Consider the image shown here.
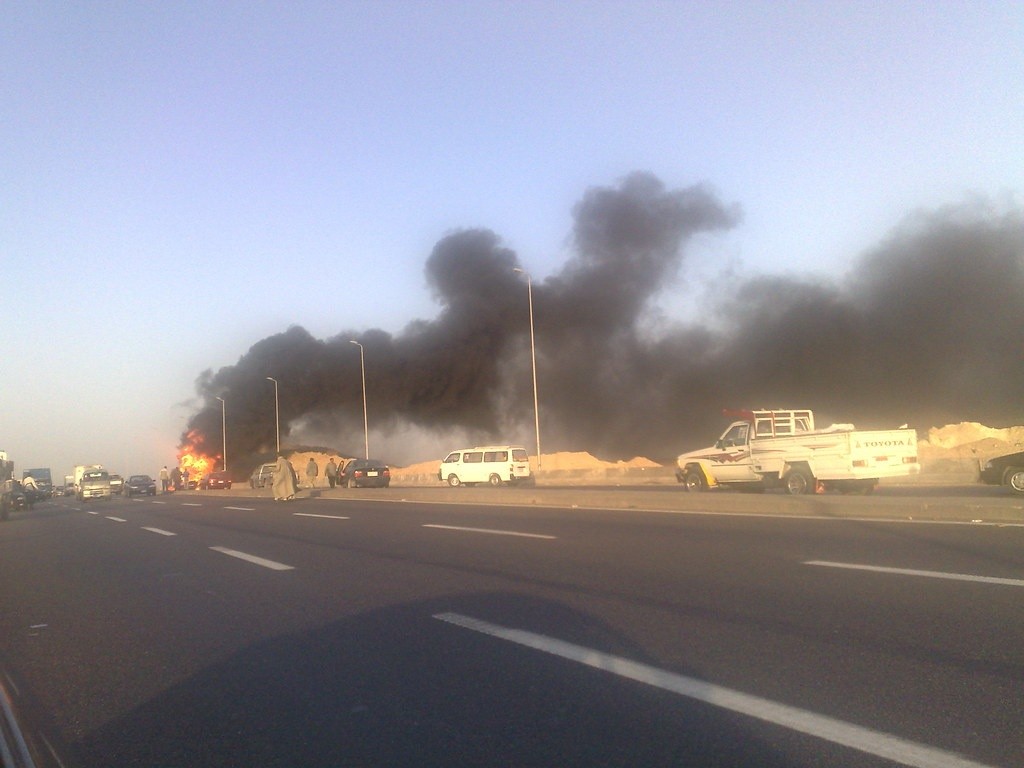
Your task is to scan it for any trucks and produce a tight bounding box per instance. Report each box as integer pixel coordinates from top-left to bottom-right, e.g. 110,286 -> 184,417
64,475 -> 76,495
250,463 -> 300,489
21,467 -> 53,499
72,464 -> 102,499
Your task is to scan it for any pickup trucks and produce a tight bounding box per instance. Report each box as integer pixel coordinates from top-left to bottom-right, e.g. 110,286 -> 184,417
670,409 -> 919,495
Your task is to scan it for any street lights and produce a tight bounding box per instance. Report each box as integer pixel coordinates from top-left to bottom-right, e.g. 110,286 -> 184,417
216,397 -> 227,472
267,377 -> 280,457
349,340 -> 368,461
514,268 -> 543,473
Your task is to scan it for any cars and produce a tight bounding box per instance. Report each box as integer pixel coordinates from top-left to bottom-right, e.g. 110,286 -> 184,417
124,474 -> 156,497
54,486 -> 66,498
980,452 -> 1024,494
199,473 -> 232,490
335,459 -> 390,488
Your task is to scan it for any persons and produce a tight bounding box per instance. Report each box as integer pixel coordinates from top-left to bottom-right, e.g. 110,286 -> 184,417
159,465 -> 189,495
324,458 -> 344,489
272,455 -> 297,501
306,458 -> 318,488
22,472 -> 38,511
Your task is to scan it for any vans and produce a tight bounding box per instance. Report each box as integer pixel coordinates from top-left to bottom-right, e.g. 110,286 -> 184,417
75,470 -> 112,502
437,446 -> 531,488
108,474 -> 124,495
